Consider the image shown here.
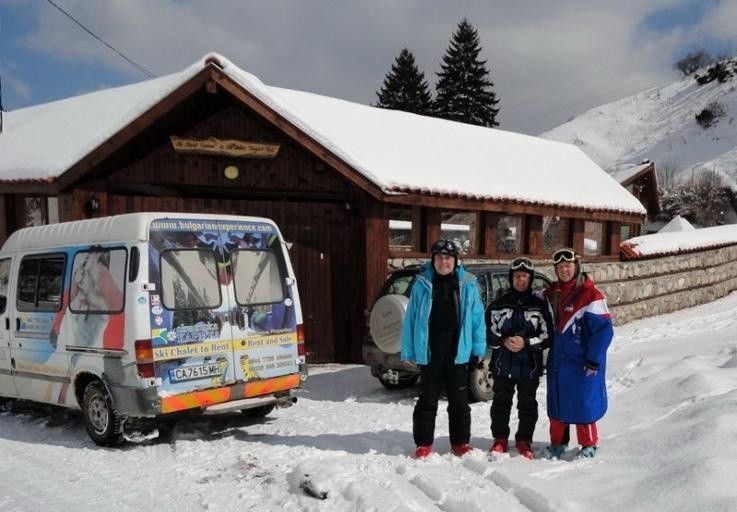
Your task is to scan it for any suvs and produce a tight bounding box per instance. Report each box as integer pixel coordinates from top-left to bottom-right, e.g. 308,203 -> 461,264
362,263 -> 552,401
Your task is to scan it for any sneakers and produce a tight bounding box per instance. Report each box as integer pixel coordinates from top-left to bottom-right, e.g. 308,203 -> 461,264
416,445 -> 432,458
545,445 -> 566,459
489,438 -> 508,453
576,445 -> 596,458
516,440 -> 535,460
454,444 -> 474,456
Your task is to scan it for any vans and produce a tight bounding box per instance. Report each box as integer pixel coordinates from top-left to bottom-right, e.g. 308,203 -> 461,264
0,210 -> 310,448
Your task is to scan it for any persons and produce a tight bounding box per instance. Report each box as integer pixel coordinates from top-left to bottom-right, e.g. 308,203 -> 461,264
539,248 -> 613,459
48,240 -> 121,404
483,256 -> 553,458
401,238 -> 488,461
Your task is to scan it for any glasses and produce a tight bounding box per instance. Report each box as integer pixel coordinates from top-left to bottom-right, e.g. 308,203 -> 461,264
510,257 -> 533,270
433,239 -> 455,251
553,249 -> 575,263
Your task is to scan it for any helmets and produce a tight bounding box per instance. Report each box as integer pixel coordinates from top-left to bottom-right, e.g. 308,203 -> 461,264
431,240 -> 458,265
508,259 -> 534,285
